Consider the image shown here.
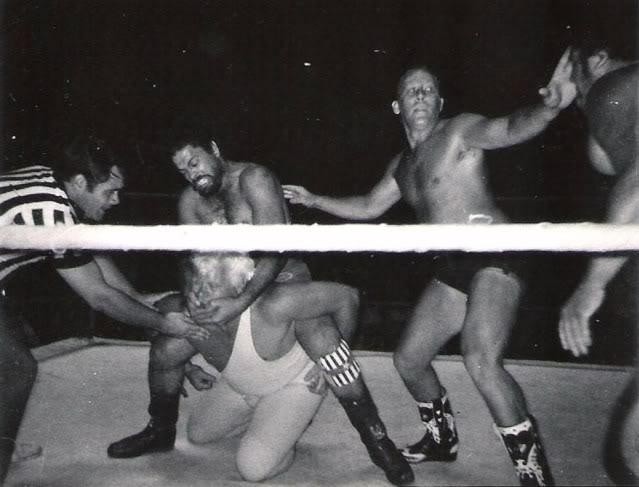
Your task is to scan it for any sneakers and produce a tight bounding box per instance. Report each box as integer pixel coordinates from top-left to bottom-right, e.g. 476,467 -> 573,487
9,443 -> 43,462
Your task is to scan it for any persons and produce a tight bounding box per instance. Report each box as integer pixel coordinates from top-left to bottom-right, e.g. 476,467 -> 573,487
106,125 -> 415,487
140,251 -> 364,483
0,132 -> 212,487
275,43 -> 582,487
555,16 -> 639,487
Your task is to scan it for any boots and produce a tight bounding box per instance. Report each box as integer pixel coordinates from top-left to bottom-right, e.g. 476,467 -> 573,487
493,417 -> 554,486
340,388 -> 414,484
399,386 -> 458,464
106,390 -> 180,457
